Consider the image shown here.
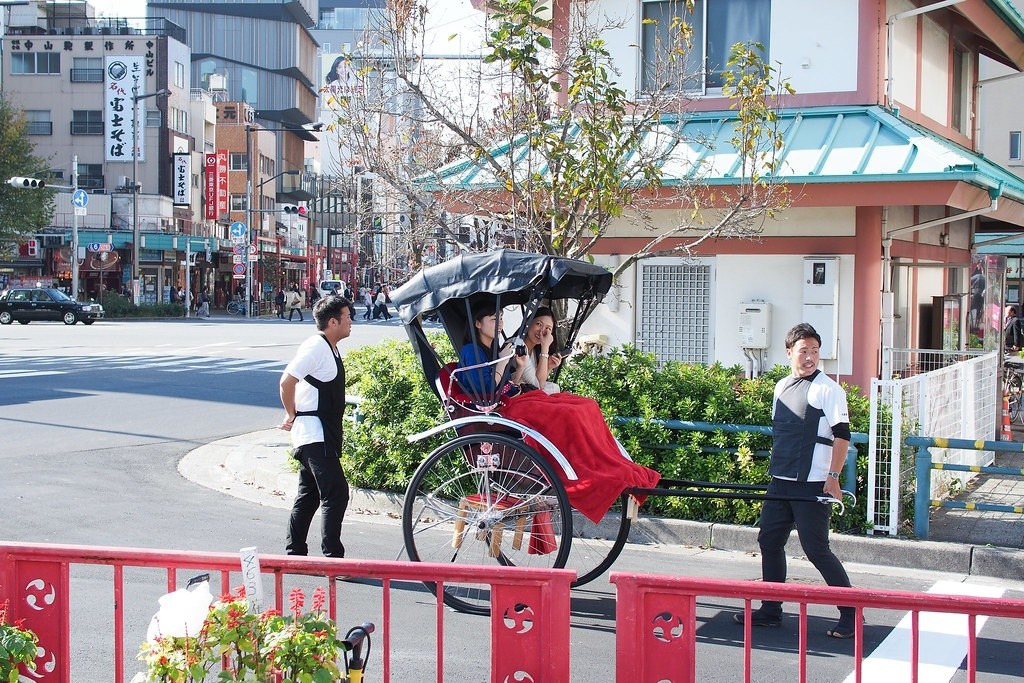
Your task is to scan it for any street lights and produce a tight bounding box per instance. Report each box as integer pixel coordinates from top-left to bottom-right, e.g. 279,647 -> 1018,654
255,168 -> 301,315
126,86 -> 171,309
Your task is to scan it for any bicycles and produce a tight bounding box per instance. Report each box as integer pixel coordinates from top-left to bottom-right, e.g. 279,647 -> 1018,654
226,295 -> 253,317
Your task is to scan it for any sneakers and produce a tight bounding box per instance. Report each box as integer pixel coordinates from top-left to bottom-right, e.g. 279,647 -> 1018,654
734,607 -> 783,628
825,607 -> 865,638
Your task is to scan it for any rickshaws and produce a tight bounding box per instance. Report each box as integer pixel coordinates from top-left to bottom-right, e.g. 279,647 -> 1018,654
381,249 -> 856,628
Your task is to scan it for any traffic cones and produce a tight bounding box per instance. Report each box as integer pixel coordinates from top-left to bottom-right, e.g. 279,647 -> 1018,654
999,387 -> 1014,443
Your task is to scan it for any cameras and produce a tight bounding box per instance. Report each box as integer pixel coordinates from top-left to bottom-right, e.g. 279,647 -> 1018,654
502,342 -> 528,357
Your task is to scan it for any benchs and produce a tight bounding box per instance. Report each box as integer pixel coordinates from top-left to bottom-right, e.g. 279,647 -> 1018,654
439,360 -> 514,420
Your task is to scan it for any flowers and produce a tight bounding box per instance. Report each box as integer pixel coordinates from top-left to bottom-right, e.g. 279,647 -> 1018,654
0,598 -> 39,682
135,575 -> 343,682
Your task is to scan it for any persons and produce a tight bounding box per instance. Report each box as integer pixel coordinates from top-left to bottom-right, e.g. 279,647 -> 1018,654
459,302 -> 528,402
504,308 -> 561,395
279,295 -> 358,581
1004,305 -> 1021,386
731,324 -> 864,638
970,259 -> 984,276
1,283 -> 404,322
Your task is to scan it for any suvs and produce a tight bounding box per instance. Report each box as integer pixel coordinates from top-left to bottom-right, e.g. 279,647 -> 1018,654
0,287 -> 105,326
370,285 -> 391,302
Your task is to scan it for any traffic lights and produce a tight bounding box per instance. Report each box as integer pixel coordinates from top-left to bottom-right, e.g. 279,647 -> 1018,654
17,178 -> 45,188
281,203 -> 305,215
26,238 -> 37,255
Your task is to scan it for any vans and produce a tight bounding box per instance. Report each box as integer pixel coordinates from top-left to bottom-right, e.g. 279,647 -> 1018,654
318,280 -> 347,300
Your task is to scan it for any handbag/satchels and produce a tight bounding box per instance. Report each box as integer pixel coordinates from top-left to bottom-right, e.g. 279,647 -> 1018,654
291,293 -> 300,305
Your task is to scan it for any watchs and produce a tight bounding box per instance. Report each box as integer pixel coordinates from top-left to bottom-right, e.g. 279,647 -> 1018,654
827,472 -> 839,480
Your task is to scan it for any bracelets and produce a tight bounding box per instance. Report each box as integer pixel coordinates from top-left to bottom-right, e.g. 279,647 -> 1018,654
539,353 -> 549,358
495,371 -> 502,379
